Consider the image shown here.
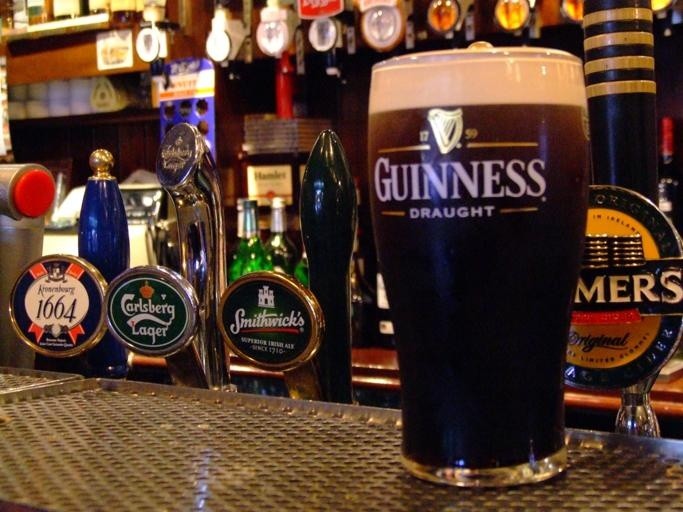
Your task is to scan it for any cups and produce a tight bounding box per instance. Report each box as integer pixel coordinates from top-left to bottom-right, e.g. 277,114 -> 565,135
366,47 -> 591,487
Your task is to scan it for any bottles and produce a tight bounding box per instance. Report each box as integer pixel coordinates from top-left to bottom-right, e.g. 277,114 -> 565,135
228,196 -> 296,288
350,176 -> 396,349
657,115 -> 683,240
